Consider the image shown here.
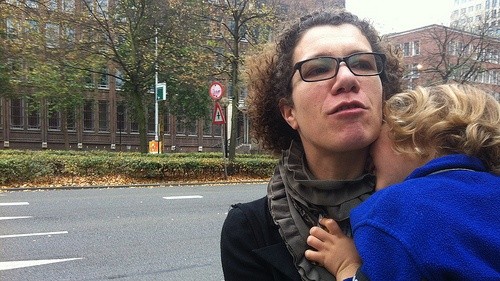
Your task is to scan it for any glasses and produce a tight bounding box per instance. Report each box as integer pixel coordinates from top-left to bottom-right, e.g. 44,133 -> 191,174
287,52 -> 386,88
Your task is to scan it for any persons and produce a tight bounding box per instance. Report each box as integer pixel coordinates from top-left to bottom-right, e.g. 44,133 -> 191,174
303,84 -> 500,281
220,7 -> 405,281
